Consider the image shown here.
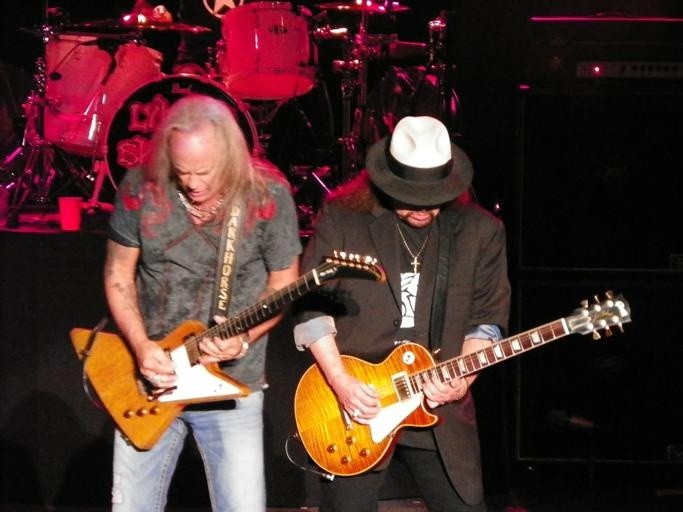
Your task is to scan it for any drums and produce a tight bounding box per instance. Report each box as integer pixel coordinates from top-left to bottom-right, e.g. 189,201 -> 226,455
43,34 -> 164,159
264,60 -> 462,230
220,1 -> 318,100
104,73 -> 260,191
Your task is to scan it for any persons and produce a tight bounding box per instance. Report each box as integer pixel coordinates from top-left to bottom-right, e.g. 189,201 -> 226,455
103,96 -> 303,512
293,116 -> 512,512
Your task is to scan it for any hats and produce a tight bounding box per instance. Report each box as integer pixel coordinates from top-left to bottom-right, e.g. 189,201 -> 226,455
364,110 -> 473,208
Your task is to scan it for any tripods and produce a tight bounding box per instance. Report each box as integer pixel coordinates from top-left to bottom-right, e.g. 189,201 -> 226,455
0,40 -> 115,228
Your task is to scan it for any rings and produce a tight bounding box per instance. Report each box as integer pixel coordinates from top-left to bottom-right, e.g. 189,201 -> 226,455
352,409 -> 359,417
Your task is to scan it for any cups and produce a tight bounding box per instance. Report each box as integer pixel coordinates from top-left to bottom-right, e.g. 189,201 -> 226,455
58,197 -> 82,231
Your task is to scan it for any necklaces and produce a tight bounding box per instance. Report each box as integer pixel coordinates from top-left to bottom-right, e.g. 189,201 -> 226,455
178,192 -> 224,218
396,223 -> 428,275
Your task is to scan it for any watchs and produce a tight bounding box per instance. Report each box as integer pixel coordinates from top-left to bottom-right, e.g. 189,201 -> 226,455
234,334 -> 249,360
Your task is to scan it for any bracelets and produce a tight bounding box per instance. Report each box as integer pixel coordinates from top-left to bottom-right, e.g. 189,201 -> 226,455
463,377 -> 469,391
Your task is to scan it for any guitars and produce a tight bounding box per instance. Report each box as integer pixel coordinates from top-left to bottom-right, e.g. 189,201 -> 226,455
294,291 -> 631,477
65,250 -> 386,451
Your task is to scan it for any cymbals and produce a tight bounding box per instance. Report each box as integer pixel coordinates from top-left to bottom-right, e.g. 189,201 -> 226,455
399,41 -> 427,48
138,23 -> 211,34
319,0 -> 409,15
